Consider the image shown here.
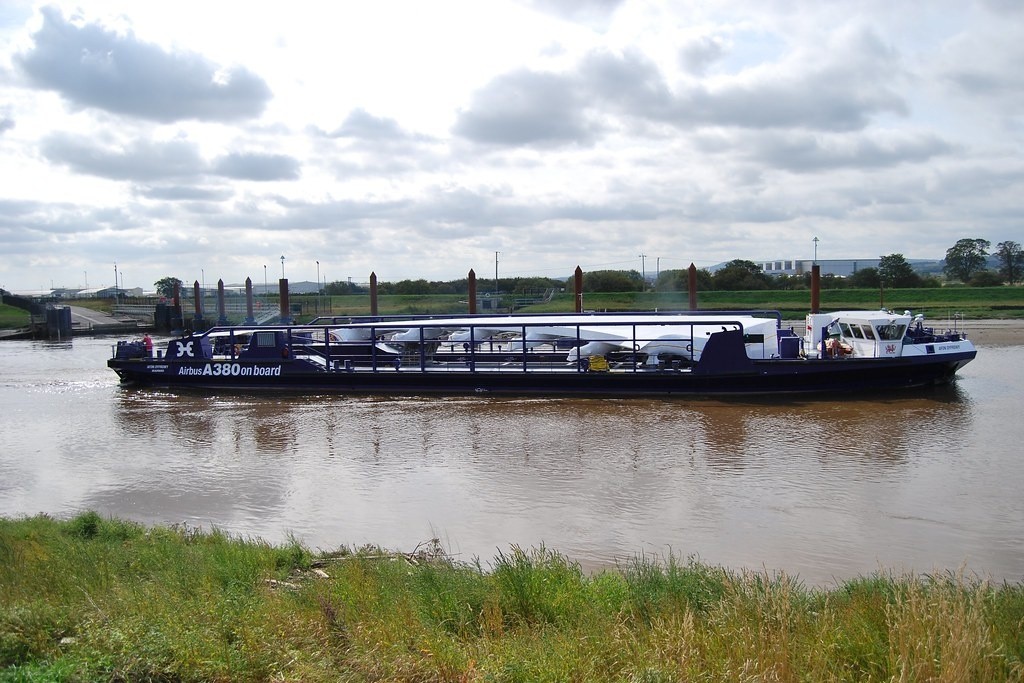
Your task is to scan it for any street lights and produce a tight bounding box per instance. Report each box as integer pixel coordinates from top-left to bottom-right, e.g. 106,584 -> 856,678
639,253 -> 647,292
316,261 -> 320,307
84,271 -> 87,301
264,265 -> 267,303
120,272 -> 124,304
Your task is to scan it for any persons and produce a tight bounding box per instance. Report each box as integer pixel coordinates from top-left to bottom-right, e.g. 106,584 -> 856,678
256,300 -> 260,311
141,333 -> 152,357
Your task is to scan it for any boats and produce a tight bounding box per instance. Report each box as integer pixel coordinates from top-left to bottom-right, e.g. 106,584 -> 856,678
106,309 -> 977,394
